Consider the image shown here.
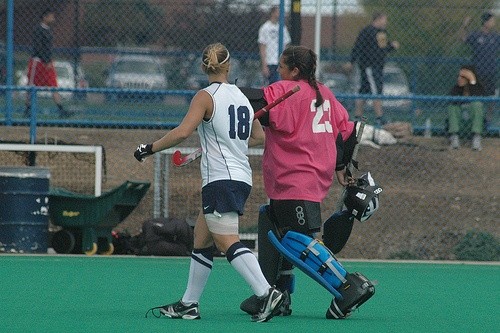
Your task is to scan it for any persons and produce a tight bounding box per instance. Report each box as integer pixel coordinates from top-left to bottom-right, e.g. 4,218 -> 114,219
342,12 -> 401,125
257,7 -> 293,84
458,13 -> 500,99
24,9 -> 74,117
238,45 -> 374,318
135,43 -> 286,322
447,67 -> 487,150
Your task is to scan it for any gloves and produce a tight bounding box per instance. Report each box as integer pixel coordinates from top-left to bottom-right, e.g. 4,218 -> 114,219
134,143 -> 155,162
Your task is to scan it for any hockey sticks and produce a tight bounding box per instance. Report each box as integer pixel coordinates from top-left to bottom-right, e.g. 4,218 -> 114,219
170,85 -> 300,167
335,116 -> 369,213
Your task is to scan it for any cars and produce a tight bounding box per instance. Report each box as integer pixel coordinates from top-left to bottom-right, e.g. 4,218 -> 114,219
183,56 -> 249,105
319,72 -> 356,115
15,60 -> 76,103
352,63 -> 414,116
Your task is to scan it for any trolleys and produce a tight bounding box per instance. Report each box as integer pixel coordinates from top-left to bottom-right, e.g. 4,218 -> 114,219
44,180 -> 152,257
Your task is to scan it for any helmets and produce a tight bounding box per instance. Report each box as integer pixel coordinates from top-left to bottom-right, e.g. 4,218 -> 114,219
347,171 -> 381,221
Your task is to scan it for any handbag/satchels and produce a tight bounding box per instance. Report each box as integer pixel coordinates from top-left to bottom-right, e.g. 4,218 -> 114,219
137,214 -> 195,257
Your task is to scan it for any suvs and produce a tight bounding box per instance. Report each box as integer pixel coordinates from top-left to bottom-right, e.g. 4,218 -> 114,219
101,53 -> 168,104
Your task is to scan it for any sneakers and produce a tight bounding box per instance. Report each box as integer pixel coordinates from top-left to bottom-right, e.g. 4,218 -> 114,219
250,286 -> 288,323
160,299 -> 202,320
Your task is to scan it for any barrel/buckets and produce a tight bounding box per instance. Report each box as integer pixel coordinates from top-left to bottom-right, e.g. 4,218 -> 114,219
0,165 -> 50,254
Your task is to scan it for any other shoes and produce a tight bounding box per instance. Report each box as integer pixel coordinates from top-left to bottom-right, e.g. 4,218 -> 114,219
58,110 -> 74,120
471,135 -> 482,152
326,271 -> 376,319
240,290 -> 292,314
450,136 -> 460,149
